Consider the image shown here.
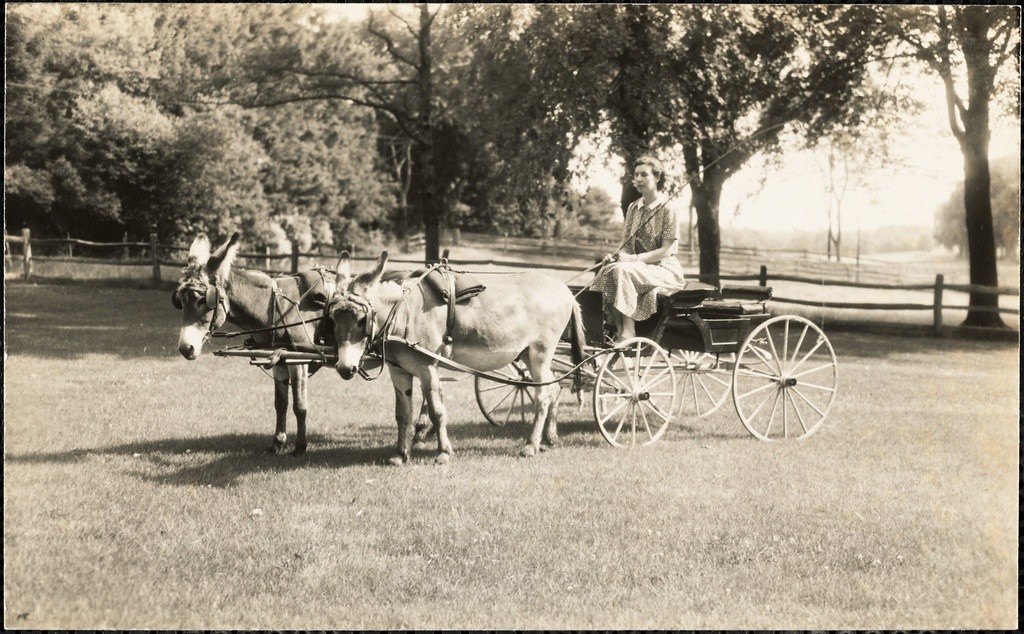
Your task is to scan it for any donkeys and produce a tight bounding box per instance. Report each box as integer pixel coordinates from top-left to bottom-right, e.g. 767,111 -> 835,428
172,231 -> 587,467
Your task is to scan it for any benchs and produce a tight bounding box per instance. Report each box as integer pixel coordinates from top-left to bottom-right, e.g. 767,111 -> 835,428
700,282 -> 773,314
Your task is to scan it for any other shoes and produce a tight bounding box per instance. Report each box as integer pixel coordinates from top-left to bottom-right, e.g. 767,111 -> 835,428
607,335 -> 636,349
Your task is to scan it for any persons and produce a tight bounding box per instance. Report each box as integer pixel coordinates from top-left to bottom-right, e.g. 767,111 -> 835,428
589,156 -> 685,346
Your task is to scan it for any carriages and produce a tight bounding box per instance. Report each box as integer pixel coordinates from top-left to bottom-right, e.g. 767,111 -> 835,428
172,227 -> 844,467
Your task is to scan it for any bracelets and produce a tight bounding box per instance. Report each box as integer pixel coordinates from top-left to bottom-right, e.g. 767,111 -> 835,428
635,254 -> 640,262
